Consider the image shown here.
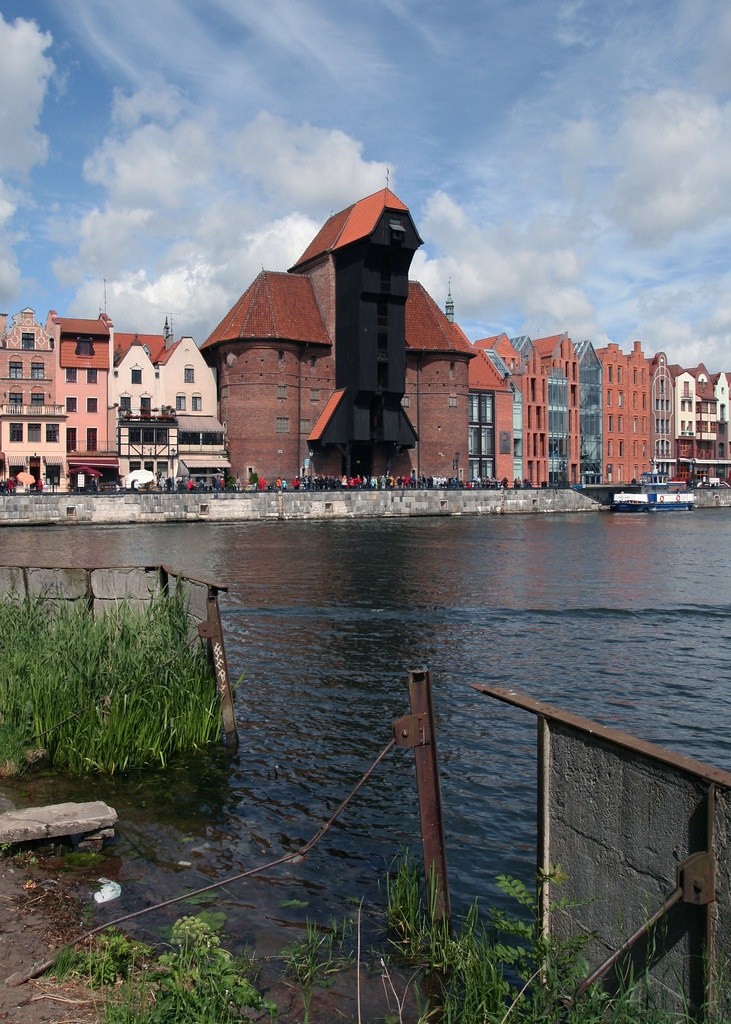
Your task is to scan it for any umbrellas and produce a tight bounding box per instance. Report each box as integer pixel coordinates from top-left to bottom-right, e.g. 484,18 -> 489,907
127,469 -> 153,484
17,472 -> 36,484
69,466 -> 101,477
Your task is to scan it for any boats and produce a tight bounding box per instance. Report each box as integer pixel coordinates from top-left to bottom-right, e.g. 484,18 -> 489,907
609,457 -> 696,511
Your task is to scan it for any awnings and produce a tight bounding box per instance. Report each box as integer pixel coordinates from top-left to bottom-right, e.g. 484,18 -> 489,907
9,456 -> 26,467
46,456 -> 63,466
68,460 -> 119,468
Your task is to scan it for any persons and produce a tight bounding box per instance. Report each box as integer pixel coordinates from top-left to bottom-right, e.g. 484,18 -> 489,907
282,479 -> 287,489
259,475 -> 265,490
177,478 -> 224,491
513,477 -> 521,488
524,479 -> 532,488
89,477 -> 99,491
1,477 -> 17,494
130,479 -> 139,491
276,477 -> 282,489
36,480 -> 43,492
166,477 -> 172,490
155,468 -> 162,486
293,474 -> 508,490
